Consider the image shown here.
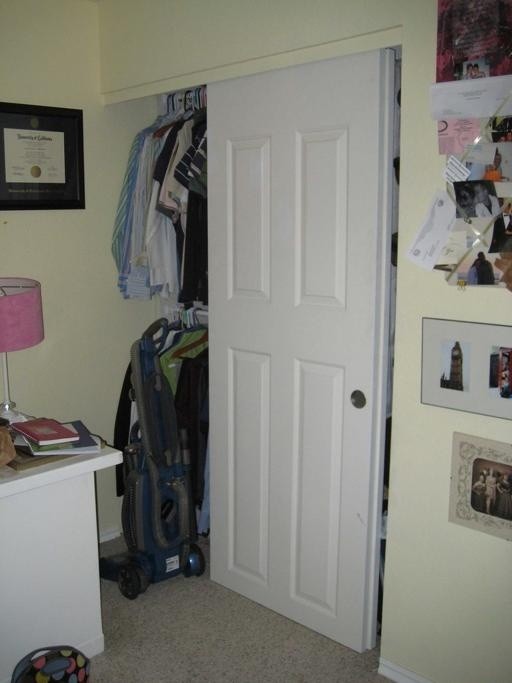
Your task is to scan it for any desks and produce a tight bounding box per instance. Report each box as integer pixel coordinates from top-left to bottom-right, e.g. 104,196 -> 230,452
0,447 -> 124,683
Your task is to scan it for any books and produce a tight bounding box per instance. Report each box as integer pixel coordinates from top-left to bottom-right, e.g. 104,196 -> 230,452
20,418 -> 99,456
11,418 -> 80,445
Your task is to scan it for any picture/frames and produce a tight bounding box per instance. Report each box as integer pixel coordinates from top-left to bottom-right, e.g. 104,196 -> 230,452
0,102 -> 85,211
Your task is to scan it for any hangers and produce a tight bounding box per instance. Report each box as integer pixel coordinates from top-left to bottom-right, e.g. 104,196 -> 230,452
146,86 -> 206,138
138,303 -> 208,361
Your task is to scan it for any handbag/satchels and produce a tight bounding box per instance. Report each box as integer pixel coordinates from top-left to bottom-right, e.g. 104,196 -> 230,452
12,646 -> 91,683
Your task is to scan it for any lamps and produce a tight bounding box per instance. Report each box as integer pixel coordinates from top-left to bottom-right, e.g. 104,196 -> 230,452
1,276 -> 44,408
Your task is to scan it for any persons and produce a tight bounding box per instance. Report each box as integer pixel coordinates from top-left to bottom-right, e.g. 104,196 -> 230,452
466,259 -> 482,284
471,63 -> 484,78
470,472 -> 486,511
488,201 -> 512,253
481,468 -> 488,482
485,466 -> 497,513
480,149 -> 510,181
473,182 -> 499,217
462,63 -> 474,78
496,471 -> 511,518
475,251 -> 495,284
